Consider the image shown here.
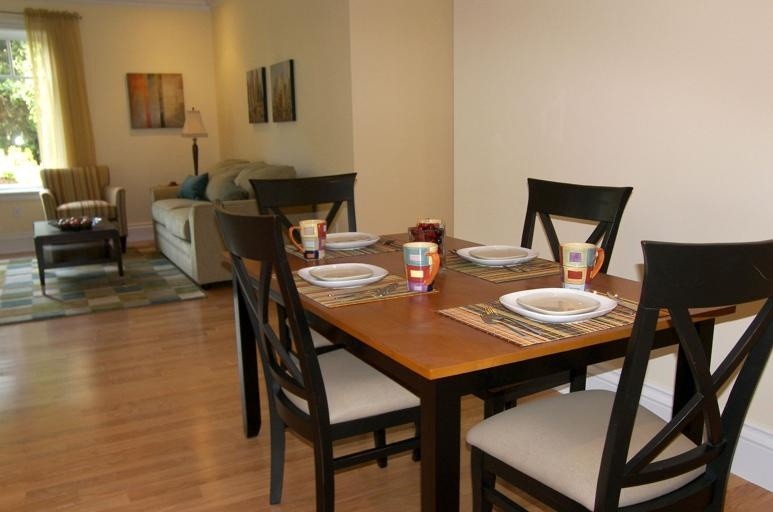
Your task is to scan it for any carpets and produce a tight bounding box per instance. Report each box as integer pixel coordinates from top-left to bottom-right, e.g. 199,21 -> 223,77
0,244 -> 207,327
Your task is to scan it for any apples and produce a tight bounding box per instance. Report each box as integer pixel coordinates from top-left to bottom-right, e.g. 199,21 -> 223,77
56,217 -> 88,226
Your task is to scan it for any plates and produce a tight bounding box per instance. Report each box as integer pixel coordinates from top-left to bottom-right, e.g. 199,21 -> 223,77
296,262 -> 388,289
469,244 -> 528,262
328,232 -> 380,253
499,285 -> 619,321
516,292 -> 600,317
307,264 -> 372,282
456,246 -> 540,269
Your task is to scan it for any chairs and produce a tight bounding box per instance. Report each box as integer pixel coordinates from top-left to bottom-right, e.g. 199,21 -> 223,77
38,163 -> 130,263
245,170 -> 372,371
464,234 -> 772,512
461,176 -> 633,424
209,204 -> 425,512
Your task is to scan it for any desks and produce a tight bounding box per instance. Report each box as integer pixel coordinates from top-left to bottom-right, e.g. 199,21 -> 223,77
218,226 -> 740,512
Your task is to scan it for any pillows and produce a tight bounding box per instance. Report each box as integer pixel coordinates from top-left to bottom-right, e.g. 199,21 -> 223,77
214,178 -> 249,203
176,171 -> 210,203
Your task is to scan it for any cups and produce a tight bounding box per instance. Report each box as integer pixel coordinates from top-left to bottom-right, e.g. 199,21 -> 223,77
555,241 -> 605,291
401,241 -> 440,294
287,219 -> 327,260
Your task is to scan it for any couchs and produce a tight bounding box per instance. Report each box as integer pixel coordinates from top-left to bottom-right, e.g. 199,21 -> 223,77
146,157 -> 315,291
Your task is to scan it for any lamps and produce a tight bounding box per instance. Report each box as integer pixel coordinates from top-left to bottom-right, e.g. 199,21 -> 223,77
179,106 -> 213,177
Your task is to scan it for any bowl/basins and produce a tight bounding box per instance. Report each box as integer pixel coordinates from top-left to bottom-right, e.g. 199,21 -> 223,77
47,217 -> 103,231
